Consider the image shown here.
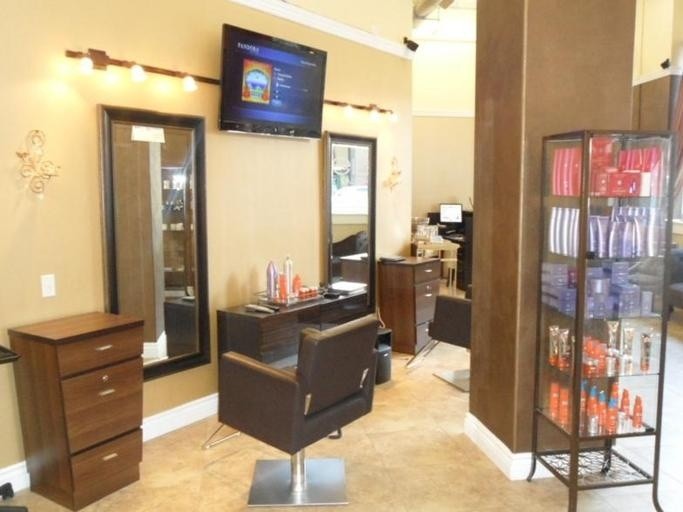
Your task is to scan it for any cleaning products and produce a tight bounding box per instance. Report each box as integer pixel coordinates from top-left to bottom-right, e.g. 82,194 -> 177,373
265,255 -> 302,302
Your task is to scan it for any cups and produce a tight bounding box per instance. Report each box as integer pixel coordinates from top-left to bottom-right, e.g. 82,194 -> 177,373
297,287 -> 318,298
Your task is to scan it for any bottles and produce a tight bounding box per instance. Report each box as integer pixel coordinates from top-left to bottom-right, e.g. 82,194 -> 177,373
617,147 -> 663,198
266,257 -> 302,299
546,207 -> 580,259
551,147 -> 581,197
546,379 -> 644,438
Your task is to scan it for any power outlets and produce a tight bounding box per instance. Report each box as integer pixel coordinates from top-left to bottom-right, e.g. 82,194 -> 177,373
40,274 -> 58,296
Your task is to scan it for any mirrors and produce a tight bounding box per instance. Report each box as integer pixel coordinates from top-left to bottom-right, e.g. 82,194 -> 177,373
99,103 -> 212,385
323,131 -> 378,312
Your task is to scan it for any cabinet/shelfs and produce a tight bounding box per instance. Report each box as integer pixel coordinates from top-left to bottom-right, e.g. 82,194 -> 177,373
5,312 -> 146,511
529,130 -> 677,512
163,295 -> 199,359
215,293 -> 369,394
376,257 -> 441,357
340,252 -> 368,285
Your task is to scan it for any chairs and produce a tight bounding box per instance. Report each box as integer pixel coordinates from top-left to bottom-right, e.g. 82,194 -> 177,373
332,230 -> 369,274
663,244 -> 683,323
216,312 -> 381,511
428,283 -> 472,395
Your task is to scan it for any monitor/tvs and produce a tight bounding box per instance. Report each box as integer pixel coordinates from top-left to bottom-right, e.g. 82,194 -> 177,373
439,203 -> 463,225
214,21 -> 327,141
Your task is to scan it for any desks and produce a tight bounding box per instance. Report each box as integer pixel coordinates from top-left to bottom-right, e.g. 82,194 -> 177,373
412,240 -> 460,295
